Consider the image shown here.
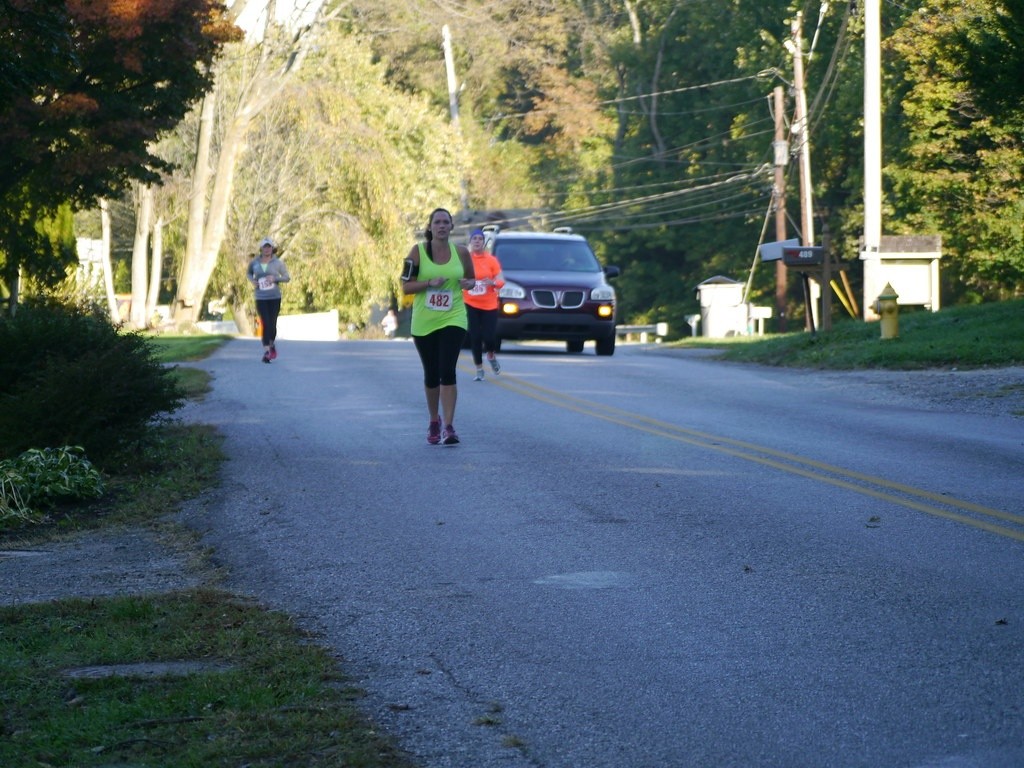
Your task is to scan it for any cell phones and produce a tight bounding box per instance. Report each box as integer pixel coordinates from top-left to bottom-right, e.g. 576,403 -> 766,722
401,259 -> 413,280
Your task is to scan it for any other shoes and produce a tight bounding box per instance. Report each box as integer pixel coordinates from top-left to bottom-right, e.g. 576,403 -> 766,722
263,351 -> 271,362
269,344 -> 276,358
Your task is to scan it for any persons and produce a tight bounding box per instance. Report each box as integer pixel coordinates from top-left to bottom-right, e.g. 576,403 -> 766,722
247,238 -> 290,364
401,208 -> 476,444
462,228 -> 506,382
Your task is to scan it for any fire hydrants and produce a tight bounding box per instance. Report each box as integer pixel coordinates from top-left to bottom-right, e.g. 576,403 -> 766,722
868,283 -> 900,340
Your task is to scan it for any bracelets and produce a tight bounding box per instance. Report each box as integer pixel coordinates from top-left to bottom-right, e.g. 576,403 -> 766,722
493,281 -> 497,286
428,279 -> 431,287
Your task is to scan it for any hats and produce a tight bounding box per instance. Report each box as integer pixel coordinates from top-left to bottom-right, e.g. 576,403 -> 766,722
261,238 -> 273,248
470,229 -> 485,243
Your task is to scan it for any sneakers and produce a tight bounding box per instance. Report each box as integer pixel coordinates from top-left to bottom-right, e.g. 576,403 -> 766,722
486,352 -> 501,374
473,369 -> 484,381
426,416 -> 442,445
441,424 -> 459,445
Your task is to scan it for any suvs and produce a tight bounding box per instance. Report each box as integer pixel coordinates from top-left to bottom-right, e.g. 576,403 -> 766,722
466,224 -> 620,355
